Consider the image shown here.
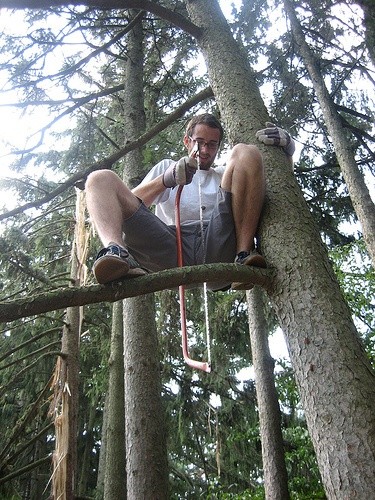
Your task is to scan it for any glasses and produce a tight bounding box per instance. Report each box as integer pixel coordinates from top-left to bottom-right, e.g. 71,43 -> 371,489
188,136 -> 219,147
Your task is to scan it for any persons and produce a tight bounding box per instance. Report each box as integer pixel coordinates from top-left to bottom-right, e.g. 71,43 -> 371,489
85,112 -> 295,290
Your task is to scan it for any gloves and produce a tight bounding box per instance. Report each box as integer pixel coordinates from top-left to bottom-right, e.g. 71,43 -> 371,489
162,156 -> 199,189
256,122 -> 296,156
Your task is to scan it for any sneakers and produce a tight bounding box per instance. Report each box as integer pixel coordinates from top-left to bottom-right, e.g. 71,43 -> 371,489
93,243 -> 145,286
231,252 -> 266,291
